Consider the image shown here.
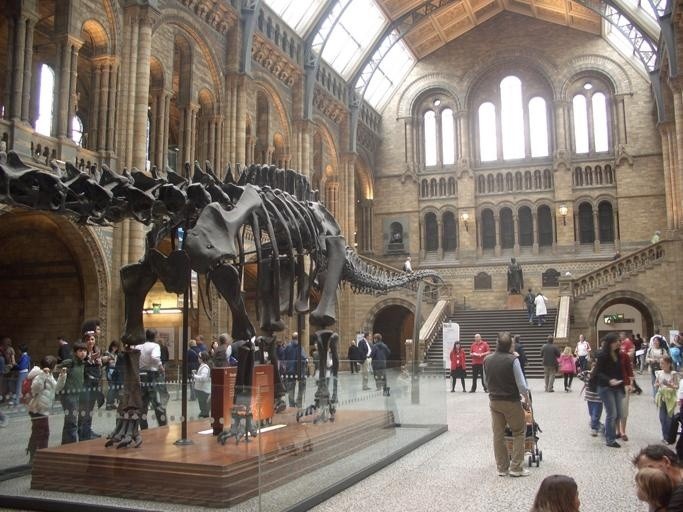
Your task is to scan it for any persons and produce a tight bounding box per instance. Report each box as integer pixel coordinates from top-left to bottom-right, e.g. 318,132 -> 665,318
523,289 -> 535,323
468,333 -> 491,393
482,332 -> 530,478
404,257 -> 414,273
533,292 -> 549,327
513,334 -> 528,382
450,341 -> 466,392
651,230 -> 662,259
614,250 -> 622,276
530,329 -> 683,512
507,256 -> 524,295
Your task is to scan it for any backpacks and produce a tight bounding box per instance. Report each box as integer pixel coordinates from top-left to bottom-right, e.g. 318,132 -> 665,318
19,373 -> 46,405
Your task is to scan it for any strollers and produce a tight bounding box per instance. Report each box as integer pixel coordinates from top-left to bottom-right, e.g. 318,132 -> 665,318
503,387 -> 542,467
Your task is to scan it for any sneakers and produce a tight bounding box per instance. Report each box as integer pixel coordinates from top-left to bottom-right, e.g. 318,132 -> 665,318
591,430 -> 598,437
606,441 -> 621,448
497,469 -> 509,476
615,432 -> 622,439
636,370 -> 643,375
509,467 -> 531,477
622,434 -> 628,442
